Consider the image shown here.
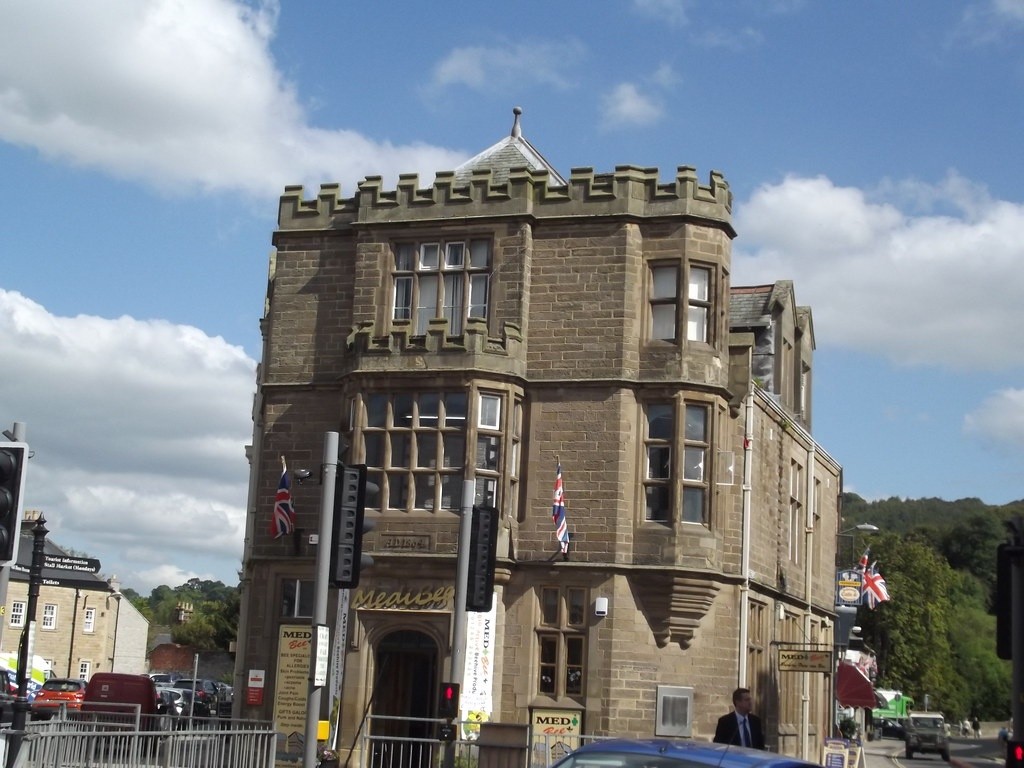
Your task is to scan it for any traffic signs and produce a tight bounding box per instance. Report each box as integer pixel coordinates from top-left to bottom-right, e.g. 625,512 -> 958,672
41,577 -> 110,592
42,552 -> 102,575
8,563 -> 31,574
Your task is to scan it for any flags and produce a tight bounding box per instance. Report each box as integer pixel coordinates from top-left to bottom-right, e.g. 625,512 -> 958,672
269,467 -> 295,538
862,562 -> 889,610
553,465 -> 570,554
855,549 -> 870,573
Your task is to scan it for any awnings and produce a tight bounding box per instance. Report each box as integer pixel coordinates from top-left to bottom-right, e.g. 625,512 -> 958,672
874,691 -> 889,709
837,662 -> 877,710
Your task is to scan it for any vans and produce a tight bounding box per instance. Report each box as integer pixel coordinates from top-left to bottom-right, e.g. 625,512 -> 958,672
80,671 -> 168,758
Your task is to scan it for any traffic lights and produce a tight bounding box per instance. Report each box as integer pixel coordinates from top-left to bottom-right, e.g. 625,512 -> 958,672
0,441 -> 30,567
327,461 -> 380,589
1005,741 -> 1024,768
437,681 -> 461,719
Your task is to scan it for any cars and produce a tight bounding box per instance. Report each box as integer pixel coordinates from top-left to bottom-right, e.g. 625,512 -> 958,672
547,735 -> 824,768
871,716 -> 904,738
0,668 -> 16,730
30,676 -> 89,721
140,672 -> 232,730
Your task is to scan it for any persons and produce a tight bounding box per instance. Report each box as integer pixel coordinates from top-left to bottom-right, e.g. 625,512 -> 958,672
713,688 -> 764,750
998,727 -> 1008,758
972,717 -> 980,739
964,718 -> 970,737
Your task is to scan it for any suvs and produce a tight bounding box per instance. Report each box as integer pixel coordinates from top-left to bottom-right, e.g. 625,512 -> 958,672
901,709 -> 952,761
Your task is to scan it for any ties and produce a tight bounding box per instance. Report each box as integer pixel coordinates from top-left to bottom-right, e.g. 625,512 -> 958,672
743,718 -> 751,748
